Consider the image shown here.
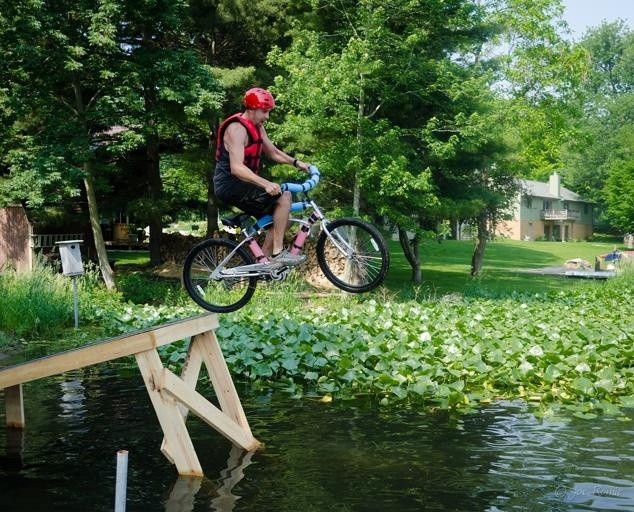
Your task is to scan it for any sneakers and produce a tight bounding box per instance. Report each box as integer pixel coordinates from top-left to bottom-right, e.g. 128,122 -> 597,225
269,248 -> 307,269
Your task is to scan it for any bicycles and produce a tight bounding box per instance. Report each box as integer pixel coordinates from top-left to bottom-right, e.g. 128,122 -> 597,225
183,162 -> 391,313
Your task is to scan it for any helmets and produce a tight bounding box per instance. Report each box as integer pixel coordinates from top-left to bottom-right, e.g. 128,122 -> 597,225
242,86 -> 275,111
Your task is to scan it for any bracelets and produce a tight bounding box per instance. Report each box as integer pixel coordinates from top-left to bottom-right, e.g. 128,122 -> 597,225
293,160 -> 297,167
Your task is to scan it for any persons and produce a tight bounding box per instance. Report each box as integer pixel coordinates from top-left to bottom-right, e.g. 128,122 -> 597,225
211,86 -> 308,281
